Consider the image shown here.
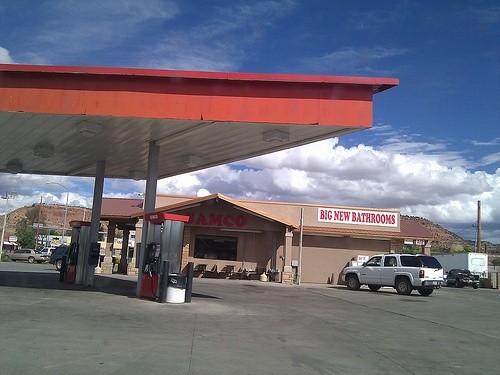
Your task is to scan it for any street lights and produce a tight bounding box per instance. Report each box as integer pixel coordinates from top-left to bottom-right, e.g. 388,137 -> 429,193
46,181 -> 69,242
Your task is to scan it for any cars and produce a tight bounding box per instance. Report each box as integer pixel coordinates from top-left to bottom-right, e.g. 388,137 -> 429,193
441,274 -> 448,286
41,247 -> 56,258
1,248 -> 48,264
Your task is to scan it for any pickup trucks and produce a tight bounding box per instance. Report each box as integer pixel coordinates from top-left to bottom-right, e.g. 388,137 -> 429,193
446,268 -> 480,289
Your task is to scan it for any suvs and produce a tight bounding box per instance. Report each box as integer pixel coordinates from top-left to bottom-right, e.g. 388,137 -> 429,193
341,253 -> 445,296
49,244 -> 69,271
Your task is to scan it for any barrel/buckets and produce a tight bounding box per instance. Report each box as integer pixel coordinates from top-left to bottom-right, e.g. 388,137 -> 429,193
166,273 -> 187,303
